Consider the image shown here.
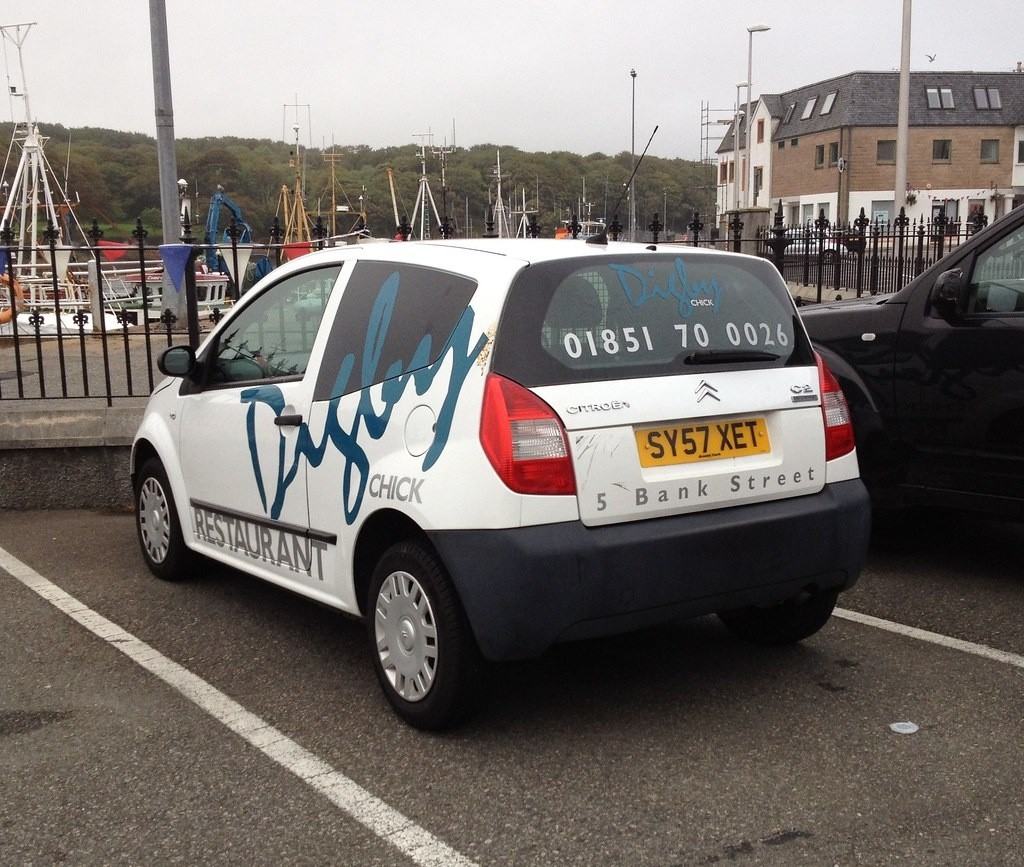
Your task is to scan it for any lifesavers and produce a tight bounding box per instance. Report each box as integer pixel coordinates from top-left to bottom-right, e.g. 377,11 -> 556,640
0,272 -> 25,325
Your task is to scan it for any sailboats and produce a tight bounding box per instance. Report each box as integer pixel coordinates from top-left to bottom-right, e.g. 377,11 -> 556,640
0,21 -> 232,338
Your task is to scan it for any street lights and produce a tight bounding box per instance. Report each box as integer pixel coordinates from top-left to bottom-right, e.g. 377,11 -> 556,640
732,81 -> 752,211
743,24 -> 771,208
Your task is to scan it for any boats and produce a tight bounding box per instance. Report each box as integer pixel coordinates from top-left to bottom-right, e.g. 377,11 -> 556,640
127,271 -> 229,311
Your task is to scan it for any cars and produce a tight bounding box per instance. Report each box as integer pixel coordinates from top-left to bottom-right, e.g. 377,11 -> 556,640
128,238 -> 874,730
795,203 -> 1024,553
